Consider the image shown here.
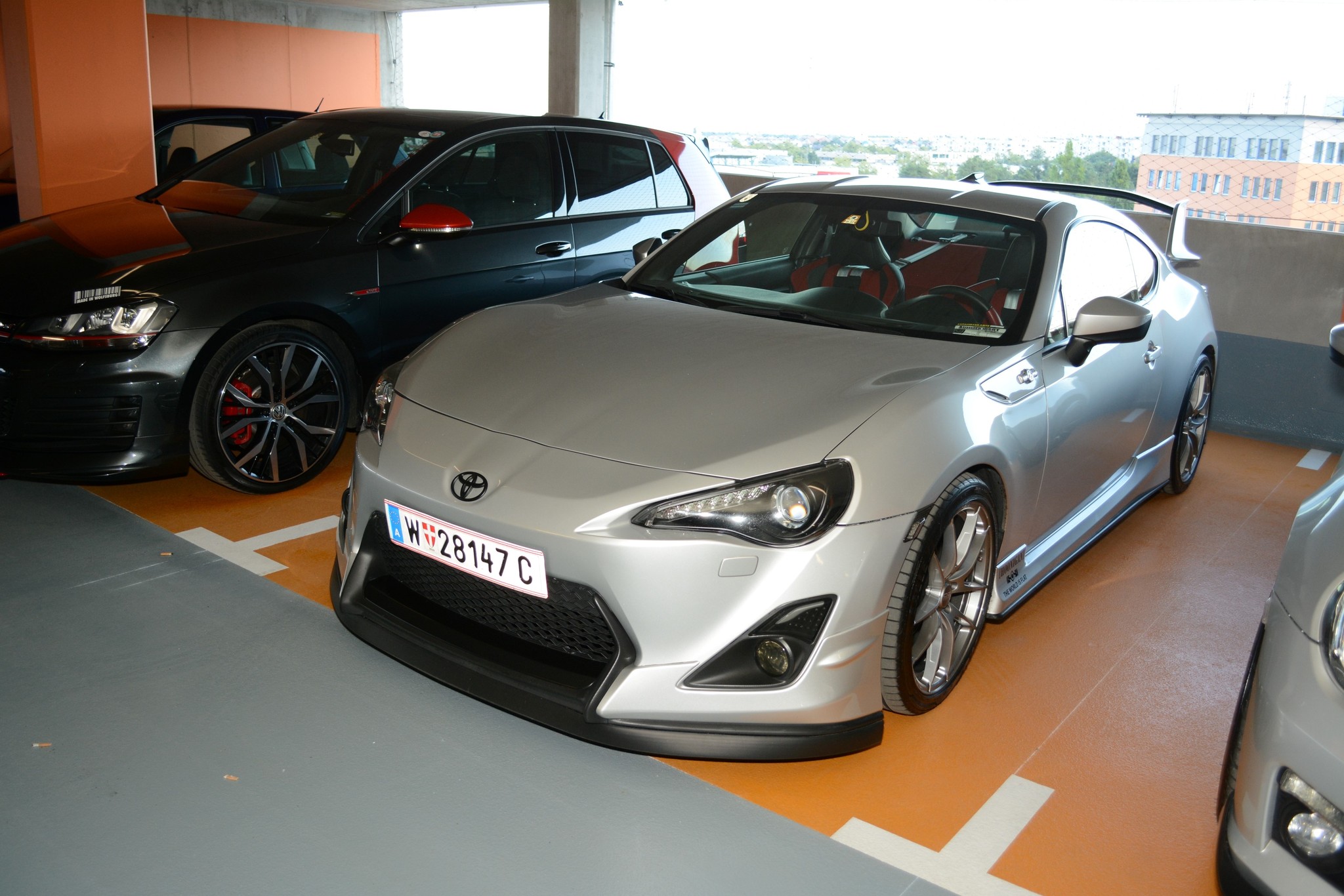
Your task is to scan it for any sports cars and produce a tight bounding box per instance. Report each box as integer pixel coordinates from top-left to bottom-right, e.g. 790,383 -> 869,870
331,170 -> 1219,765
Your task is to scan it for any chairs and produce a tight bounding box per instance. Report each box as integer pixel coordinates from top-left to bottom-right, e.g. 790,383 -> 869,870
789,217 -> 906,307
951,236 -> 1033,324
308,145 -> 350,181
166,147 -> 198,179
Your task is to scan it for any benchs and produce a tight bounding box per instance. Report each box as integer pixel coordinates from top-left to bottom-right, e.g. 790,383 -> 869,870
898,239 -> 1007,325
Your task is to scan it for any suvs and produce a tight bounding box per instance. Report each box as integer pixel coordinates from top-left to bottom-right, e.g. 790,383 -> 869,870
0,105 -> 751,496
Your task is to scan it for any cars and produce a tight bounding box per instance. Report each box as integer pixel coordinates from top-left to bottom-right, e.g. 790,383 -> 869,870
1209,318 -> 1344,896
